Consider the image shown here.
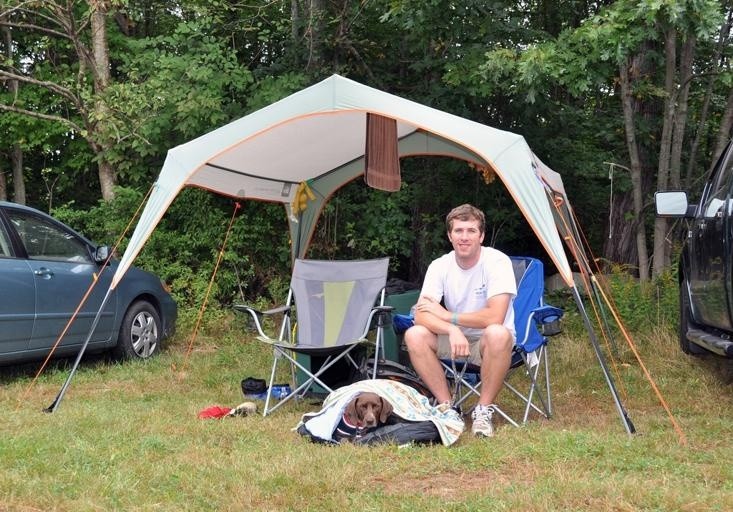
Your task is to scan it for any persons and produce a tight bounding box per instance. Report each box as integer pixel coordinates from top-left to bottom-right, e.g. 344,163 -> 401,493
404,204 -> 517,439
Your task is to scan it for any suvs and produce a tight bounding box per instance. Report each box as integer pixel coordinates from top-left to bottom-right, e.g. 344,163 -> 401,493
651,135 -> 732,368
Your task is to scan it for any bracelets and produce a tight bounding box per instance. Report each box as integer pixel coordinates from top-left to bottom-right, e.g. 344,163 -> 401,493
450,312 -> 457,326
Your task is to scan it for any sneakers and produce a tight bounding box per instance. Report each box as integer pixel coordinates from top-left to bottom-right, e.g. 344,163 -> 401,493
470,405 -> 495,438
438,400 -> 466,430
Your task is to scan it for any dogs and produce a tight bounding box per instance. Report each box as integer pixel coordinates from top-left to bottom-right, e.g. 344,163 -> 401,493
330,392 -> 393,446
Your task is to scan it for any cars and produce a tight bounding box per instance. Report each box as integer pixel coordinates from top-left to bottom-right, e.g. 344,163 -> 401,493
0,200 -> 180,370
